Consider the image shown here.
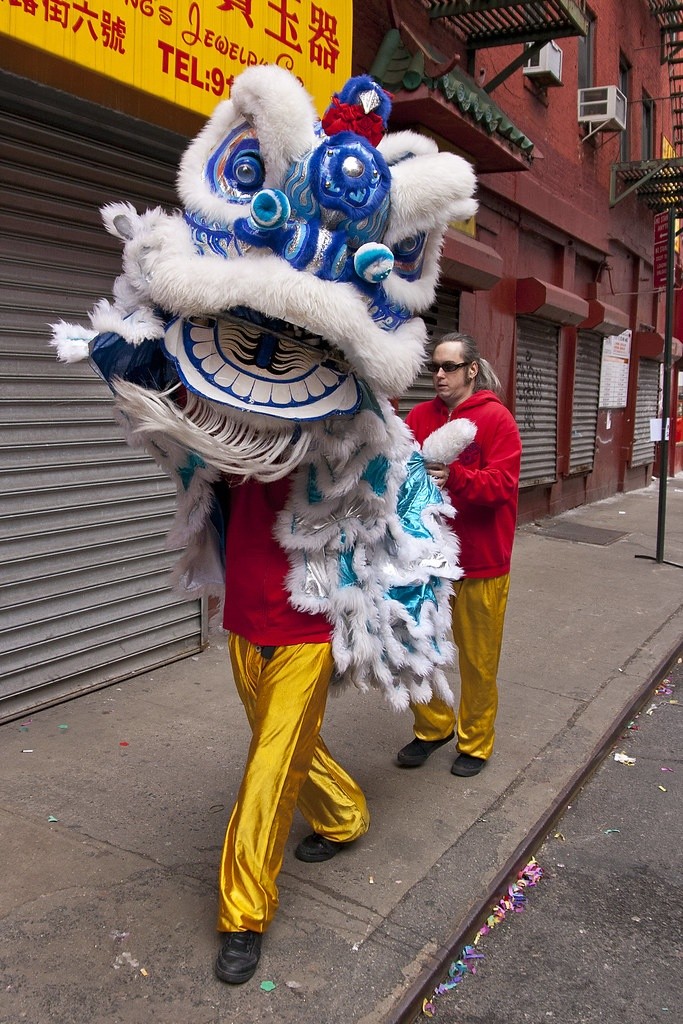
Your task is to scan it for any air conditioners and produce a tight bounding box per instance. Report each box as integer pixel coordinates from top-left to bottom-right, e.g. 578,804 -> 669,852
578,86 -> 627,132
523,40 -> 563,87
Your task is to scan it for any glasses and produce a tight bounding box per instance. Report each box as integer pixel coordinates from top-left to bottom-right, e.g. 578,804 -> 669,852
427,361 -> 474,373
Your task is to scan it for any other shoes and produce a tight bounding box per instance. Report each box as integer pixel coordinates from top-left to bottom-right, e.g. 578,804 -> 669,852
451,752 -> 487,776
295,830 -> 354,863
215,931 -> 262,984
397,730 -> 455,767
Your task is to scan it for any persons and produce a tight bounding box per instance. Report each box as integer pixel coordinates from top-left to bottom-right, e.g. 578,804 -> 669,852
394,331 -> 522,776
212,438 -> 373,985
